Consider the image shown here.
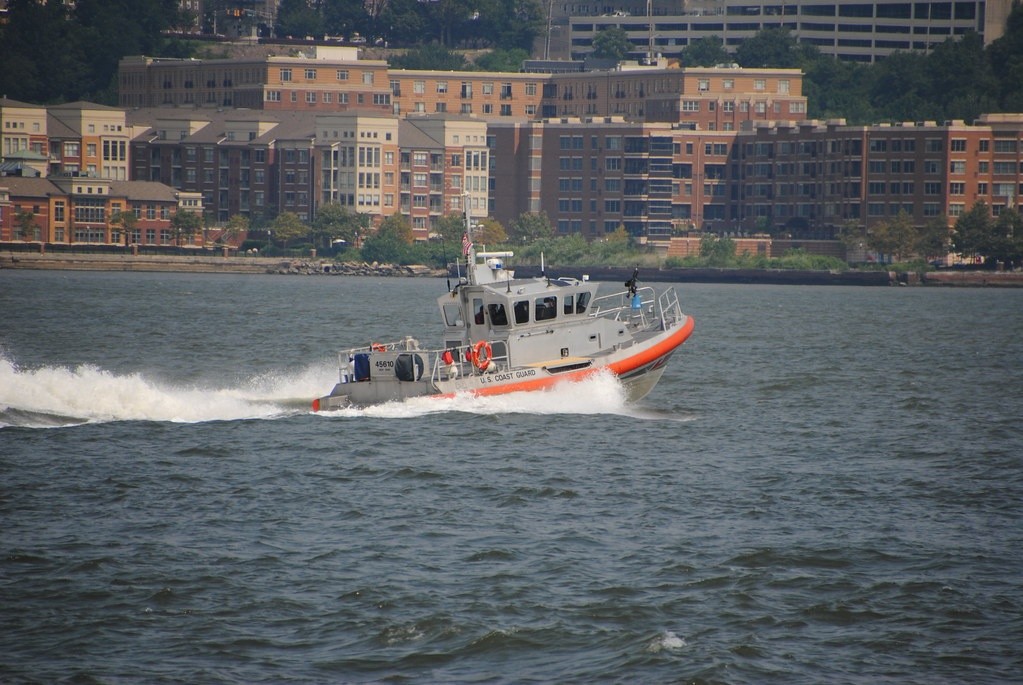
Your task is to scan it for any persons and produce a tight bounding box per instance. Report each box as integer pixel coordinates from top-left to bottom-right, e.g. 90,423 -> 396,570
475,304 -> 528,325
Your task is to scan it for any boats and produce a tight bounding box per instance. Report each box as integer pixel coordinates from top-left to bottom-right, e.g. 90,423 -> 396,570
311,191 -> 694,413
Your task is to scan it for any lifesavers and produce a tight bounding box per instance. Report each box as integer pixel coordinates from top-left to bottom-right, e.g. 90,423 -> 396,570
473,340 -> 492,369
372,343 -> 387,353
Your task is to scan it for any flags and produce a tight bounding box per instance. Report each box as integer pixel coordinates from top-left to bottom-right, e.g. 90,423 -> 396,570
462,233 -> 473,255
